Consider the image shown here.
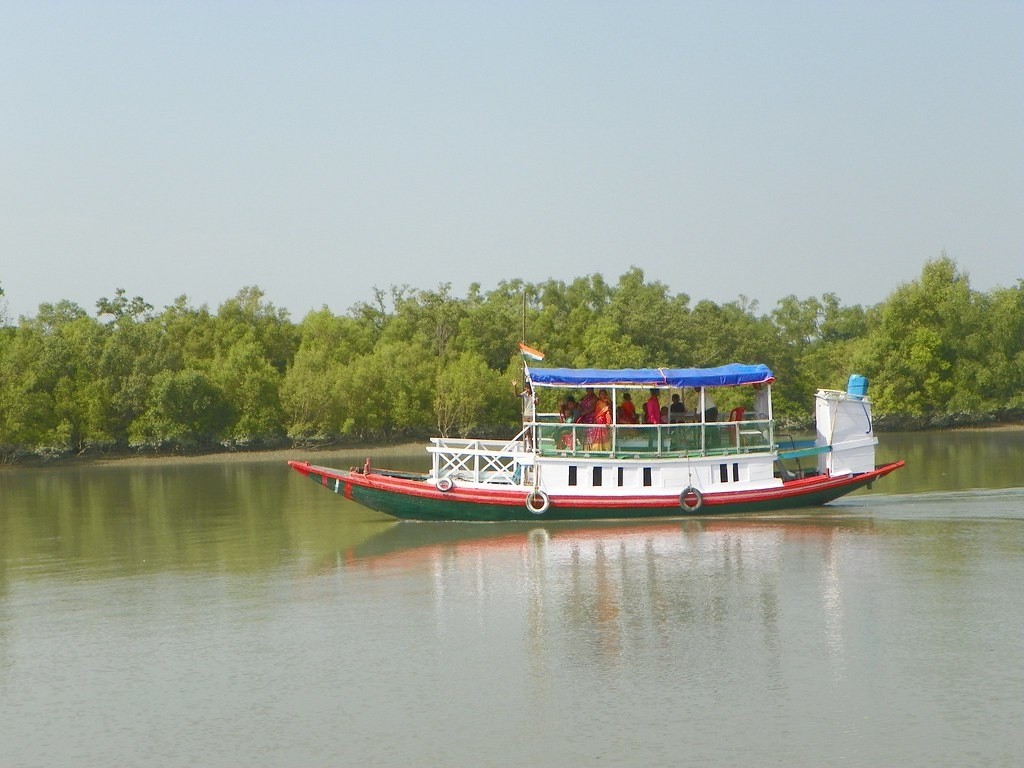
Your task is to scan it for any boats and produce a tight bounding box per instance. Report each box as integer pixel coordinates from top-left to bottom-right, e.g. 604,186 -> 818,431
286,290 -> 906,524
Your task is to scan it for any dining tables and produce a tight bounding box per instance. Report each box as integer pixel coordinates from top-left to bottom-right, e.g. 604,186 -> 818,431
671,411 -> 697,445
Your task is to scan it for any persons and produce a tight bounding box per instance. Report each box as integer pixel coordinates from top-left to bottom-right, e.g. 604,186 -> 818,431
557,400 -> 581,450
595,389 -> 614,452
580,388 -> 599,452
552,410 -> 573,443
511,378 -> 540,453
622,393 -> 637,437
559,395 -> 581,422
660,406 -> 672,438
670,394 -> 687,447
693,385 -> 723,449
615,406 -> 622,438
637,403 -> 648,439
648,389 -> 666,451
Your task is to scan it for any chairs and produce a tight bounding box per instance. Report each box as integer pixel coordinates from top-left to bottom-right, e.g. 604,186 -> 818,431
718,407 -> 744,446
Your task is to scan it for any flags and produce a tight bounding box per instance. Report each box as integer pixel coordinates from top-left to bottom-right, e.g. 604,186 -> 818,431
519,341 -> 546,363
